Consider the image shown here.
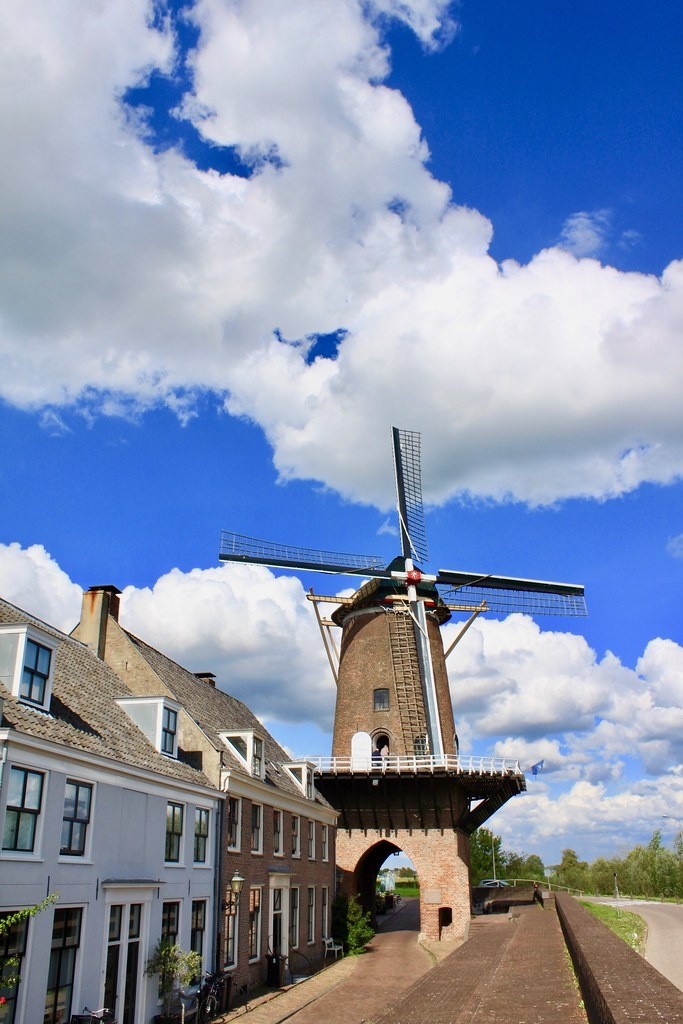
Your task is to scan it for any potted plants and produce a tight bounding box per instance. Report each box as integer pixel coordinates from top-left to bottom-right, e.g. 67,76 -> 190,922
142,939 -> 188,1024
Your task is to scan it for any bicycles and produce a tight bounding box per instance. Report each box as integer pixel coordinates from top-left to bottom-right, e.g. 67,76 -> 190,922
83,1006 -> 119,1024
197,970 -> 232,1024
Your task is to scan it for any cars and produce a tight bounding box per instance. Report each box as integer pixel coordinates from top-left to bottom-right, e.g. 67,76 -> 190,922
478,879 -> 514,887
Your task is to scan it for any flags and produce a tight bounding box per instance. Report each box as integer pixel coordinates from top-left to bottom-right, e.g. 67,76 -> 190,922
531,760 -> 546,780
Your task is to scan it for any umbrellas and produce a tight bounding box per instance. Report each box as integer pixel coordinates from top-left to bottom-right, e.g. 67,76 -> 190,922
379,874 -> 385,893
384,872 -> 394,891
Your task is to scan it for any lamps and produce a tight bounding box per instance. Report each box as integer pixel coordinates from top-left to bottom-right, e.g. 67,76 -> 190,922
223,868 -> 246,911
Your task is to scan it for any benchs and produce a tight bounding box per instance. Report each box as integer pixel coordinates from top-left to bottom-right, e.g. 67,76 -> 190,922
324,937 -> 344,959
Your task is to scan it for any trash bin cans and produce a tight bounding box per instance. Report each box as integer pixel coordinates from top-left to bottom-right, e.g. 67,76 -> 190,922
264,954 -> 288,987
